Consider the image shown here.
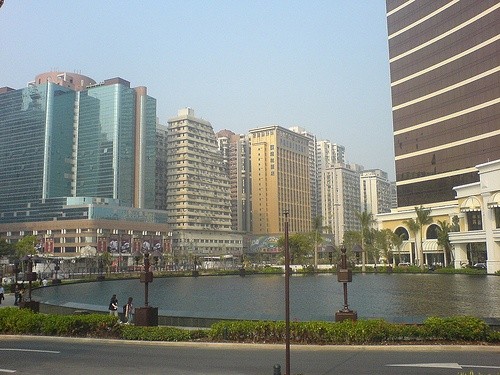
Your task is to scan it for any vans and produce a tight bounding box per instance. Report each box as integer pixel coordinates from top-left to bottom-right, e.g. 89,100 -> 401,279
398,263 -> 410,267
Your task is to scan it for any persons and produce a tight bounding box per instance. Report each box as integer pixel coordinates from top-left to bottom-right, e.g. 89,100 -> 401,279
437,260 -> 442,267
428,264 -> 434,271
42,277 -> 48,286
125,296 -> 133,322
0,285 -> 4,304
15,284 -> 24,305
109,295 -> 118,316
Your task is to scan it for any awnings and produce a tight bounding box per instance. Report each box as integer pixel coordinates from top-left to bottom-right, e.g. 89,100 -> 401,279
422,240 -> 444,254
389,242 -> 409,252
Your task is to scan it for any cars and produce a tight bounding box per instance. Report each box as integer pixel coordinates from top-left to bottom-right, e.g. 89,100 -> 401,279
473,263 -> 486,269
